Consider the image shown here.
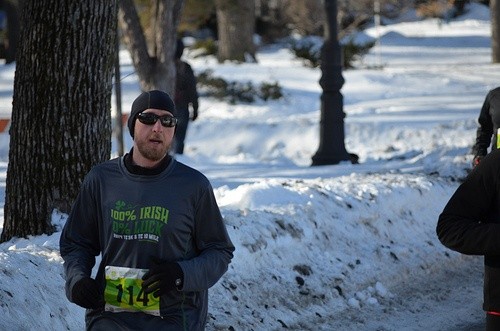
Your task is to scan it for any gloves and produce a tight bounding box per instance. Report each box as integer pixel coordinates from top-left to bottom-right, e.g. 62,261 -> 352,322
192,110 -> 197,121
141,257 -> 184,298
471,153 -> 486,170
71,277 -> 106,310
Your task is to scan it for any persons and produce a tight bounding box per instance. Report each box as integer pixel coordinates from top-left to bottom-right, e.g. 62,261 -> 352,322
472,86 -> 500,168
170,37 -> 198,154
436,148 -> 500,331
60,91 -> 236,331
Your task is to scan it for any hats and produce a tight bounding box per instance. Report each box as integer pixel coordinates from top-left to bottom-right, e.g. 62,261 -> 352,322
177,38 -> 184,50
128,90 -> 176,141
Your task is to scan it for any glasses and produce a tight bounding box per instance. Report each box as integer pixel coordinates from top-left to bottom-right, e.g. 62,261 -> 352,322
136,112 -> 178,127
